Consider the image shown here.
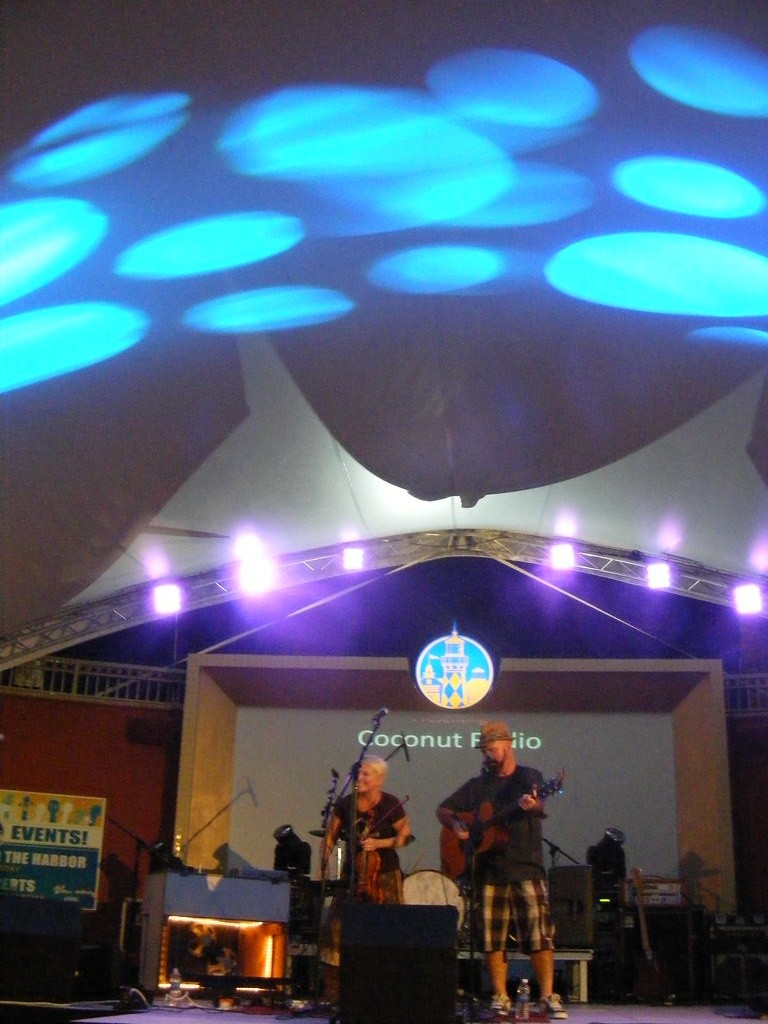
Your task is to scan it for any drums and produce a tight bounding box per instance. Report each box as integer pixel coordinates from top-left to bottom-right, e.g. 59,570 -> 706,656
403,870 -> 467,931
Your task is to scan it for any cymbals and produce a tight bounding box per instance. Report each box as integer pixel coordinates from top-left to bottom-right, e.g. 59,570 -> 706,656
308,829 -> 349,838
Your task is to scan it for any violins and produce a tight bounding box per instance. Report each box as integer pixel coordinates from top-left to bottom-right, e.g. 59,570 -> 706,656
356,808 -> 384,904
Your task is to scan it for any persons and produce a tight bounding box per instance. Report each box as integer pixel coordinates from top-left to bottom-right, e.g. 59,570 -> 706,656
435,723 -> 569,1020
316,753 -> 412,1000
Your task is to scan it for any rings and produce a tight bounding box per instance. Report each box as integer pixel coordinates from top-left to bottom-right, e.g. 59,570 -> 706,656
367,846 -> 370,848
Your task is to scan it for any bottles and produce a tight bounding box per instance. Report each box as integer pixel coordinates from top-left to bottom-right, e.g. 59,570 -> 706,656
516,979 -> 530,1019
170,968 -> 182,1005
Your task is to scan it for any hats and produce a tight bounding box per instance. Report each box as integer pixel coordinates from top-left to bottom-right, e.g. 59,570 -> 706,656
474,721 -> 515,749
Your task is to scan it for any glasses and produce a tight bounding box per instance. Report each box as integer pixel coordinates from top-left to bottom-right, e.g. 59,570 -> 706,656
480,734 -> 508,742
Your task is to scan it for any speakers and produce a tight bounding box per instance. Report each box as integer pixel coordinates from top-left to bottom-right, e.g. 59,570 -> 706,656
339,903 -> 459,1024
550,865 -> 592,950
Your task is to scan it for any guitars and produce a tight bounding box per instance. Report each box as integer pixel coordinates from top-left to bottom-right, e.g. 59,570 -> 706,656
439,765 -> 565,880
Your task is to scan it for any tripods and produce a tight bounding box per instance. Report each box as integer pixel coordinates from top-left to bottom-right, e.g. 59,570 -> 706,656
458,777 -> 514,1024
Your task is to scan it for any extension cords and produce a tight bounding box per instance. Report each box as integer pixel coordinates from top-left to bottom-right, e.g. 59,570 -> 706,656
114,996 -> 153,1011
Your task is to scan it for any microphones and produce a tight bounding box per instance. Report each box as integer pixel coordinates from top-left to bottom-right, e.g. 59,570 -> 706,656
482,751 -> 495,773
370,708 -> 389,720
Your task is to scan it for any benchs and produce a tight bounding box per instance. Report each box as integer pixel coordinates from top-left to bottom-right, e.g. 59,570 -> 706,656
456,948 -> 592,1003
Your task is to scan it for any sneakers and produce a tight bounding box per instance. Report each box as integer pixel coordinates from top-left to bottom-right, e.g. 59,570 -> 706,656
539,994 -> 568,1019
490,992 -> 512,1016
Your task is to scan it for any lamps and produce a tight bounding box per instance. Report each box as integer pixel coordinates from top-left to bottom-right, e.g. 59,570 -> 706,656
273,824 -> 311,875
587,827 -> 627,890
